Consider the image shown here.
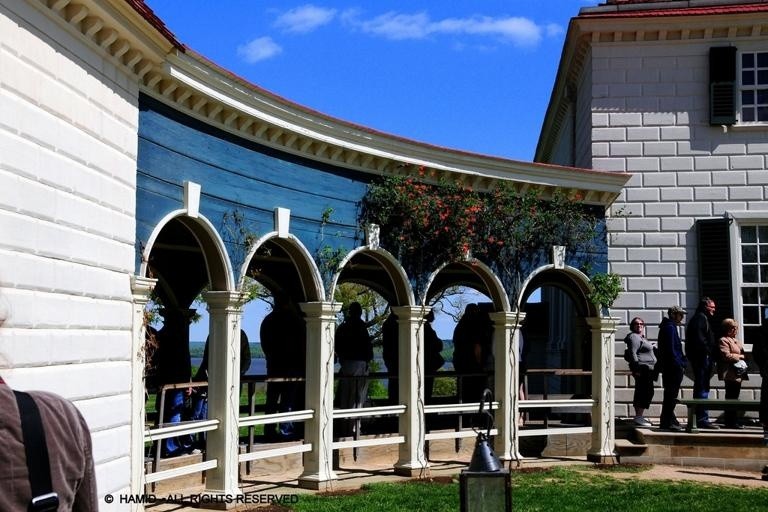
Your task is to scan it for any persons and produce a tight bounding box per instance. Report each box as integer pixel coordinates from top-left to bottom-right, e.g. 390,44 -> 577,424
623,316 -> 657,425
752,321 -> 768,429
148,300 -> 524,459
0,293 -> 100,511
656,305 -> 689,430
716,318 -> 750,428
684,295 -> 722,429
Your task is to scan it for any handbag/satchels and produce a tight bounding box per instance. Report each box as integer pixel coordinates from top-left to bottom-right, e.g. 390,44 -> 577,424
732,360 -> 748,375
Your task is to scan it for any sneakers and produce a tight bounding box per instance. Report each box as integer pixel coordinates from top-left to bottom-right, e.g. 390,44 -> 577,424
634,415 -> 651,426
698,422 -> 745,429
661,418 -> 687,432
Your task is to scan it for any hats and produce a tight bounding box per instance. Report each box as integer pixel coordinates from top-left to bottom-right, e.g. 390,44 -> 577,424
669,306 -> 688,315
